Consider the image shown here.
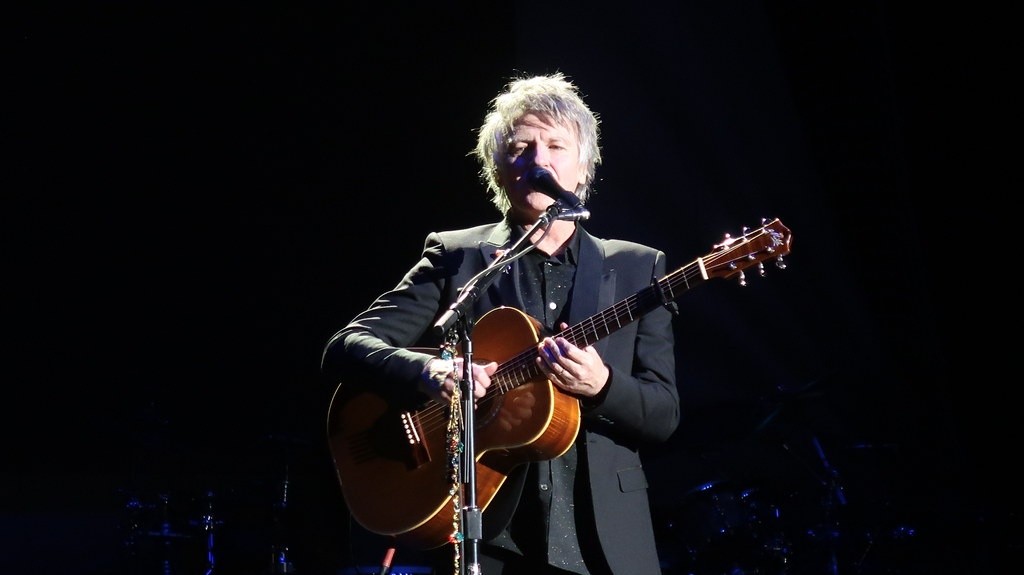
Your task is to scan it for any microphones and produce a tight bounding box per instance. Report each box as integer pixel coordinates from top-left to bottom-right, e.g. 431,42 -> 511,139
519,166 -> 589,221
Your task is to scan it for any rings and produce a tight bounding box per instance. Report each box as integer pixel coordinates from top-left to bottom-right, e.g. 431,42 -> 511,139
558,367 -> 565,376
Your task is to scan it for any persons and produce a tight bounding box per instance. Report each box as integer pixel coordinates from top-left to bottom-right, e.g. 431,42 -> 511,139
317,73 -> 681,575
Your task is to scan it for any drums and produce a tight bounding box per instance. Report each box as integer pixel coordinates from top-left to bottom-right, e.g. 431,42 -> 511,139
126,489 -> 216,541
668,477 -> 747,554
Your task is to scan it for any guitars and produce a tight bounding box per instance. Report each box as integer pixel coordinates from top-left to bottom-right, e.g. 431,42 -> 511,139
324,216 -> 793,551
